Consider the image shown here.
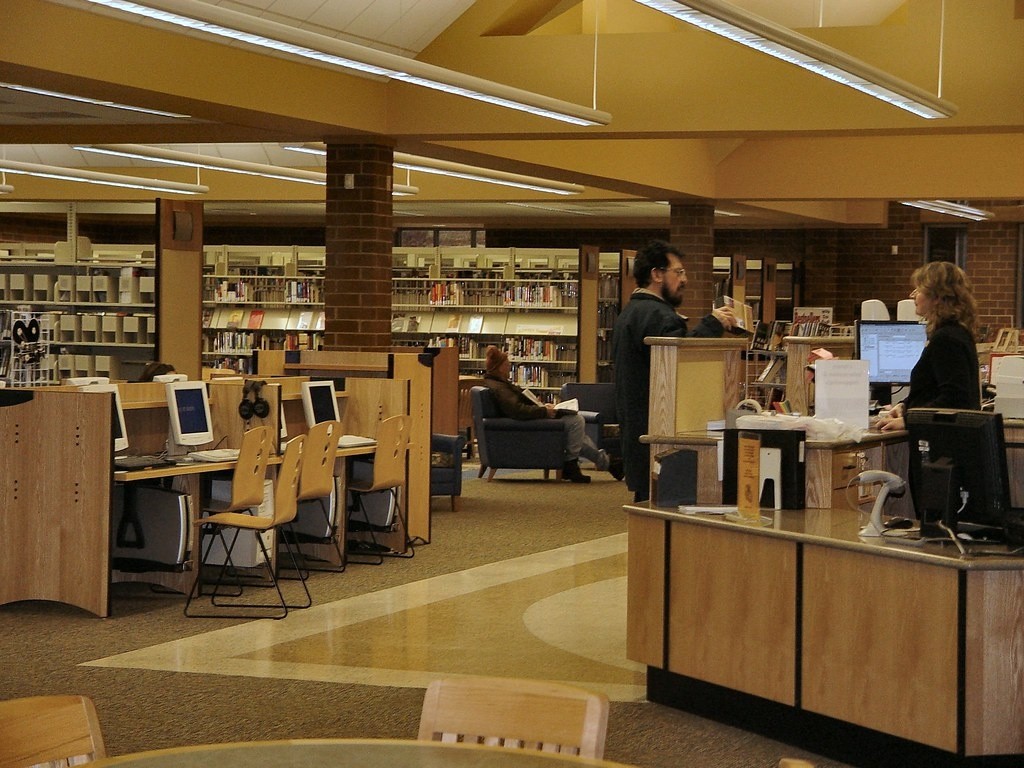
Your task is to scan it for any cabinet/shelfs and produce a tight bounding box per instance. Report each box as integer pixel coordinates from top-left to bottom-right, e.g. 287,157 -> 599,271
0,196 -> 205,386
202,245 -> 803,454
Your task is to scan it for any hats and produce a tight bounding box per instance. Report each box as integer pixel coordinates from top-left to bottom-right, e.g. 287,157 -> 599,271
486,345 -> 508,372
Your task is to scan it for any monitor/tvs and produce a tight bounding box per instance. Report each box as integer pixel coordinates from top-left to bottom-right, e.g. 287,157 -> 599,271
904,406 -> 1013,544
152,373 -> 214,462
64,376 -> 129,459
301,381 -> 340,429
854,320 -> 928,414
280,403 -> 288,438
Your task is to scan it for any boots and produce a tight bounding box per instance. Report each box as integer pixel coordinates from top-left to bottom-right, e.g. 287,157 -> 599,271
561,458 -> 591,483
608,458 -> 625,481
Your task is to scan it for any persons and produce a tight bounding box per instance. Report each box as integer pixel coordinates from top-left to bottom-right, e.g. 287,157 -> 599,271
138,362 -> 176,383
610,238 -> 737,505
875,261 -> 981,521
482,344 -> 625,484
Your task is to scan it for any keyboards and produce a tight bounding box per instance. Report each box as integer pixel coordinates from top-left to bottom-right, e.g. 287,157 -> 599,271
113,456 -> 170,472
337,435 -> 378,448
279,441 -> 303,454
188,448 -> 241,462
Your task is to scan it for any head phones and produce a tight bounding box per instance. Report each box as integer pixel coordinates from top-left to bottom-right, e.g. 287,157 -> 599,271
253,381 -> 270,418
238,380 -> 255,420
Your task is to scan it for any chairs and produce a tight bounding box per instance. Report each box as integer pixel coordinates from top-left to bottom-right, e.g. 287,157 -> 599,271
469,385 -> 568,482
276,419 -> 346,581
430,432 -> 467,512
183,432 -> 313,621
0,692 -> 108,768
417,672 -> 610,761
344,411 -> 415,565
560,381 -> 625,471
148,425 -> 277,597
458,377 -> 484,458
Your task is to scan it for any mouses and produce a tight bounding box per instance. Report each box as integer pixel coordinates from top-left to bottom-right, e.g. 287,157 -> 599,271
163,459 -> 178,466
883,516 -> 913,529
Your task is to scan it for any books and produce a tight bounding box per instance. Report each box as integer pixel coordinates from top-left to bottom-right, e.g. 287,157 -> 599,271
722,295 -> 855,411
391,269 -> 580,333
201,272 -> 326,375
520,388 -> 578,414
428,333 -> 578,405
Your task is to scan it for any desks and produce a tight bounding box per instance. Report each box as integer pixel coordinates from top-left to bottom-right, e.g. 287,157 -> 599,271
0,348 -> 436,622
74,737 -> 636,768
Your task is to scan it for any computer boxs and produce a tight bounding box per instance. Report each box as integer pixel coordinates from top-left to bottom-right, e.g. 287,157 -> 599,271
346,459 -> 401,533
199,478 -> 276,567
112,483 -> 195,573
279,474 -> 340,544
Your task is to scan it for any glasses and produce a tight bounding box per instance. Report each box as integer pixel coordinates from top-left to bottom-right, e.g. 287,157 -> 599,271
652,268 -> 685,277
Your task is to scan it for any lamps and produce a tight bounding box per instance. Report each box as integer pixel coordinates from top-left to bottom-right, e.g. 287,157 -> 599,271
277,141 -> 587,195
632,0 -> 959,121
53,0 -> 614,128
0,143 -> 210,195
899,199 -> 996,222
69,142 -> 420,196
